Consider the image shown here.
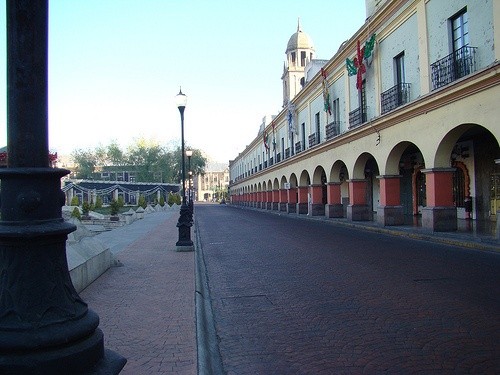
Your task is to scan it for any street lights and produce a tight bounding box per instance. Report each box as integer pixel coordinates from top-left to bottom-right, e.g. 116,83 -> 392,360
184,148 -> 193,208
173,86 -> 194,252
187,170 -> 193,204
